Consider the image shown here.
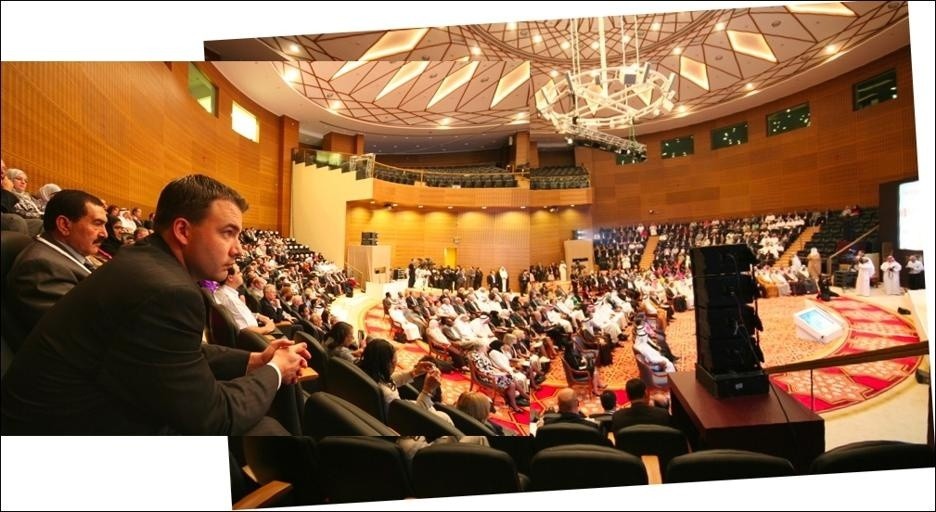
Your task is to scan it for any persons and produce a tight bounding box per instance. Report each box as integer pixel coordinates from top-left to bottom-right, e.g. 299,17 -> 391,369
0,163 -> 860,433
904,255 -> 925,289
1,172 -> 311,500
877,255 -> 902,294
852,250 -> 875,296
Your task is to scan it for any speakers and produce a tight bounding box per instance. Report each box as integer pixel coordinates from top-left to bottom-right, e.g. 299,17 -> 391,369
361,239 -> 378,246
362,232 -> 378,239
690,244 -> 769,399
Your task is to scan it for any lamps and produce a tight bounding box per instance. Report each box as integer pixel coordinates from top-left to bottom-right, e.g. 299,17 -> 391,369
540,14 -> 676,129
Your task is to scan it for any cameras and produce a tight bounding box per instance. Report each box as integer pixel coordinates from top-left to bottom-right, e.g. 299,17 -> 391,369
430,365 -> 441,376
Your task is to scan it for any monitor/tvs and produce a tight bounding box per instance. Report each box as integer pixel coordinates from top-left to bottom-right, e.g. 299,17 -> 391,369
794,306 -> 845,343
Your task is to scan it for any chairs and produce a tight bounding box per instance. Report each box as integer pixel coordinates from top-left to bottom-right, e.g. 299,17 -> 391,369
292,151 -> 589,190
1,206 -> 936,510
